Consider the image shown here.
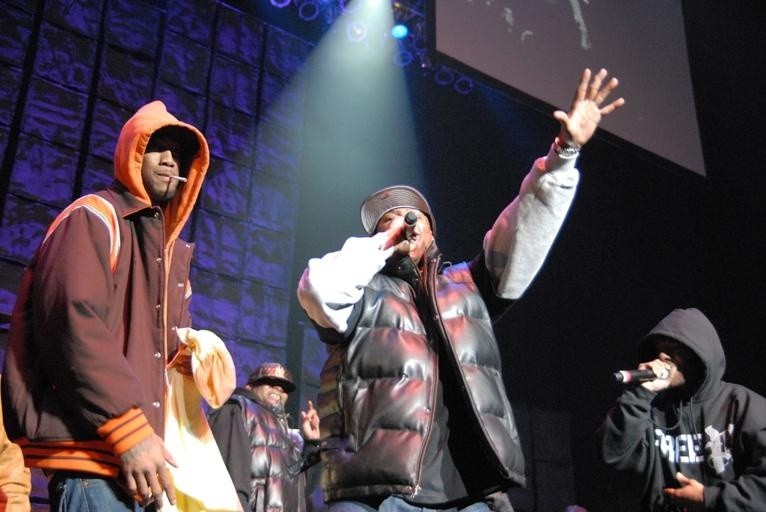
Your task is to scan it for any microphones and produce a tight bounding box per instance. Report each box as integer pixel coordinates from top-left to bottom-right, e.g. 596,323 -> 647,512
612,368 -> 656,386
404,212 -> 417,240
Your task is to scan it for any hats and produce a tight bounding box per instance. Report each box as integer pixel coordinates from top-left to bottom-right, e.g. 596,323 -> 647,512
360,184 -> 436,236
249,361 -> 296,392
